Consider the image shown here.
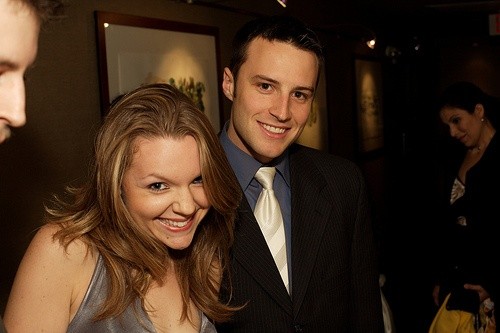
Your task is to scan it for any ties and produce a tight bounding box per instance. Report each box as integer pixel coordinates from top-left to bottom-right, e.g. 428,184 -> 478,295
253,166 -> 291,295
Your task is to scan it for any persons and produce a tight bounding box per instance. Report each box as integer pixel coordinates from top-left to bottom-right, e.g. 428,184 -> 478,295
1,83 -> 252,333
0,0 -> 41,144
400,80 -> 497,333
177,24 -> 383,332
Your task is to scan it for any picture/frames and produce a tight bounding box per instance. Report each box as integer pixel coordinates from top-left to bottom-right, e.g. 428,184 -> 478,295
95,10 -> 226,140
351,54 -> 389,163
294,62 -> 331,154
